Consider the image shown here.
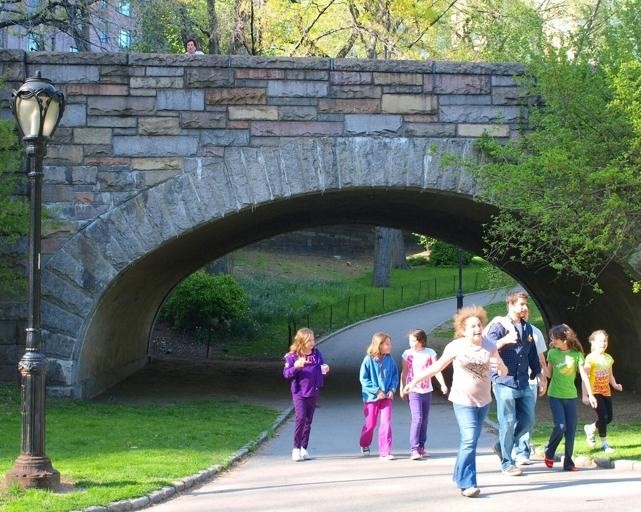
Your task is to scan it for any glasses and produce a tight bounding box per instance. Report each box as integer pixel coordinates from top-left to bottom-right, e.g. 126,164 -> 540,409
549,336 -> 560,341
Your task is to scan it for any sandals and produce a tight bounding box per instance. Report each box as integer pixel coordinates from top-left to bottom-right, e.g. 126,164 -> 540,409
544,453 -> 553,467
568,466 -> 577,471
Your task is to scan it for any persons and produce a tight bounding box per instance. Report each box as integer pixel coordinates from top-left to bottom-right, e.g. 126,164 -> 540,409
401,303 -> 510,498
282,327 -> 331,462
542,324 -> 599,472
481,290 -> 549,477
512,302 -> 549,466
359,331 -> 401,460
399,329 -> 449,460
581,329 -> 625,454
185,39 -> 205,55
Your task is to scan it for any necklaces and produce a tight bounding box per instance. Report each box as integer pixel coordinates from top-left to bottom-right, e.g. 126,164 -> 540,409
560,347 -> 571,351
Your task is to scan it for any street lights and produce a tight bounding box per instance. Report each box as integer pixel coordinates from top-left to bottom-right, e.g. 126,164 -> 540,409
7,70 -> 65,490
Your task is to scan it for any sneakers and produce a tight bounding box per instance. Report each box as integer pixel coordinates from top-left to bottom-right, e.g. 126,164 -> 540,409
410,450 -> 421,459
292,447 -> 304,461
602,442 -> 611,452
462,487 -> 480,497
380,455 -> 394,460
301,447 -> 310,460
493,443 -> 531,476
584,425 -> 596,445
361,446 -> 370,456
418,448 -> 430,457
